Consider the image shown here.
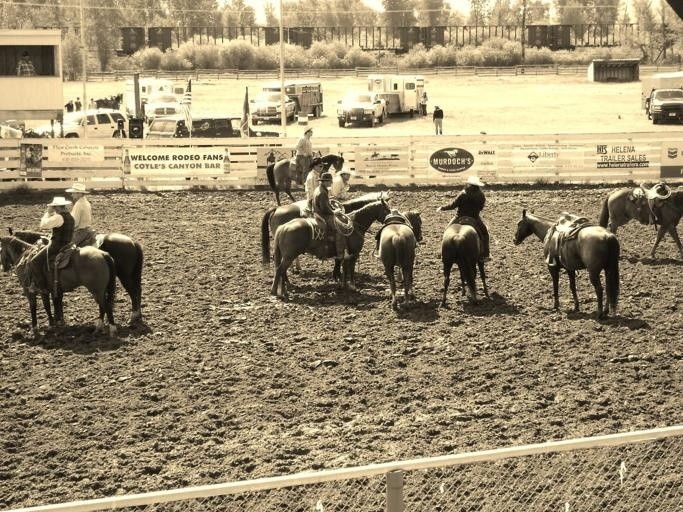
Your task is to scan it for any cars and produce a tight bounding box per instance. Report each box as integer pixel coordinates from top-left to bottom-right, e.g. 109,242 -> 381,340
0,120 -> 26,140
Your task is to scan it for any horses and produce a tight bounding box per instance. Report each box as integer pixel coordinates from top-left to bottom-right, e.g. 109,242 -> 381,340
376,208 -> 425,311
437,222 -> 490,309
265,151 -> 345,206
0,235 -> 120,339
93,93 -> 124,110
598,185 -> 683,261
260,188 -> 392,302
512,208 -> 621,321
8,226 -> 143,328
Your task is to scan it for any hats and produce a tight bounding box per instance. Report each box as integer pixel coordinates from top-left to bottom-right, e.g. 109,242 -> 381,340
462,176 -> 485,186
303,125 -> 312,133
308,158 -> 355,181
47,197 -> 72,206
65,183 -> 89,193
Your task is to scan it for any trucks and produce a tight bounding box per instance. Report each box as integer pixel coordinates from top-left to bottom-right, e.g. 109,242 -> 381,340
122,78 -> 173,117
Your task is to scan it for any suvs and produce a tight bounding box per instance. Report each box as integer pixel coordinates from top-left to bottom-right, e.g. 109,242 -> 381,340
247,92 -> 295,124
141,92 -> 180,127
643,87 -> 683,126
146,116 -> 281,168
334,92 -> 386,129
34,108 -> 128,140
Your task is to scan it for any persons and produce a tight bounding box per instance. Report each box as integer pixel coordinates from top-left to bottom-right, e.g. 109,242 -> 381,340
65,100 -> 73,112
433,106 -> 443,135
420,92 -> 428,116
435,176 -> 492,263
16,50 -> 38,76
294,125 -> 355,279
64,182 -> 92,246
88,98 -> 97,110
30,196 -> 75,293
75,97 -> 81,111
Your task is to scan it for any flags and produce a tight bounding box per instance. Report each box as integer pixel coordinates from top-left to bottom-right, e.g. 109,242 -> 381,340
240,87 -> 250,137
180,79 -> 192,132
